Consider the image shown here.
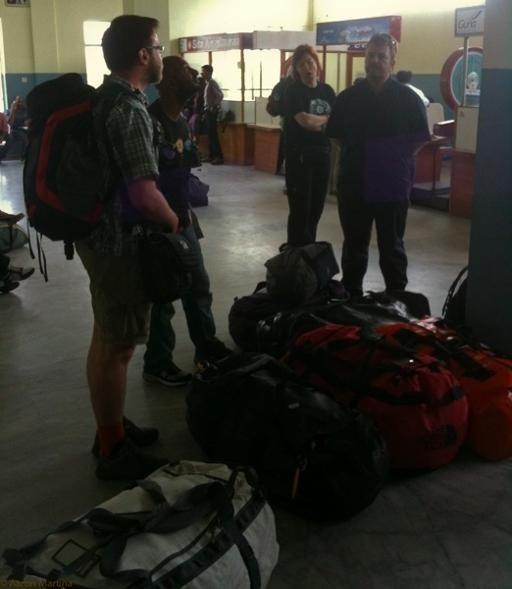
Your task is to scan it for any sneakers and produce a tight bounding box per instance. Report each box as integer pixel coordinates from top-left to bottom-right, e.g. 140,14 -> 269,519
0,210 -> 24,224
0,265 -> 35,294
142,356 -> 191,386
91,415 -> 178,481
200,156 -> 224,165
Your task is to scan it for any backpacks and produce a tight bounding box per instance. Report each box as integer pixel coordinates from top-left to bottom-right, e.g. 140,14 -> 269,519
22,72 -> 137,241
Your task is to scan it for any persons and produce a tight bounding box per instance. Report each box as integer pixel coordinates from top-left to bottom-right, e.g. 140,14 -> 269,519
77,14 -> 181,484
397,67 -> 429,207
142,57 -> 233,388
199,64 -> 229,165
8,95 -> 29,159
185,78 -> 203,143
281,45 -> 336,244
0,111 -> 10,162
326,33 -> 430,299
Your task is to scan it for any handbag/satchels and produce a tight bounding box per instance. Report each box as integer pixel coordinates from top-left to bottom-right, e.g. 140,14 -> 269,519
185,352 -> 388,524
279,320 -> 469,469
257,292 -> 405,359
0,460 -> 281,589
264,242 -> 339,305
228,279 -> 349,352
369,315 -> 512,461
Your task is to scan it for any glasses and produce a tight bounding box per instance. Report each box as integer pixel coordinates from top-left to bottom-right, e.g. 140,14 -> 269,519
145,45 -> 165,53
